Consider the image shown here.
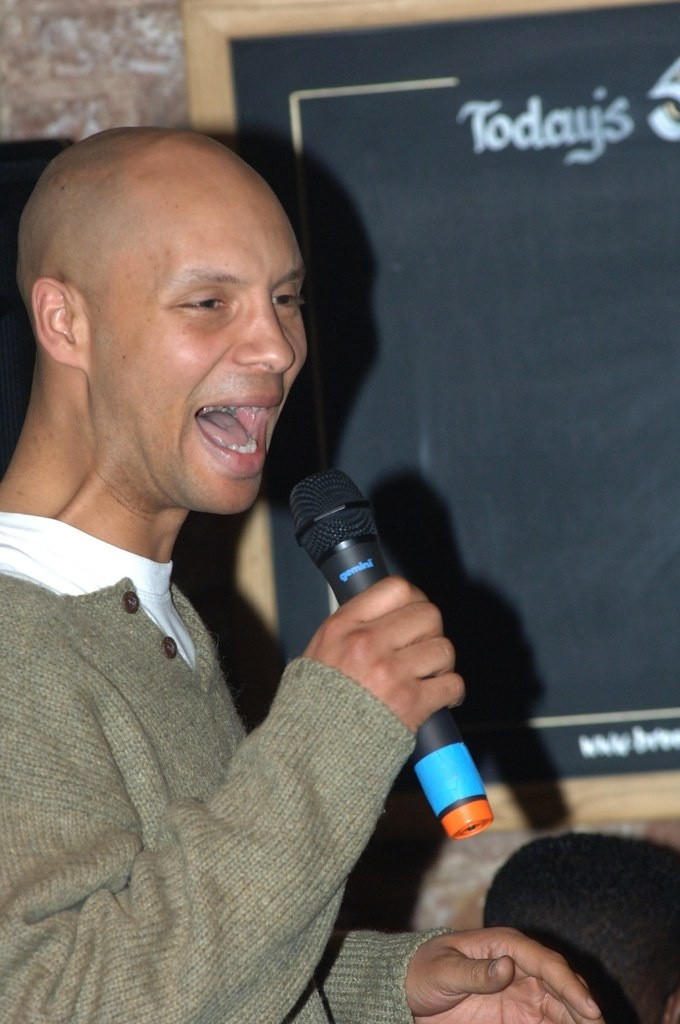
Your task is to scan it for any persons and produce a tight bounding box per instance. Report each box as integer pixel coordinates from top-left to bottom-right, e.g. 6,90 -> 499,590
483,831 -> 680,1024
0,125 -> 607,1024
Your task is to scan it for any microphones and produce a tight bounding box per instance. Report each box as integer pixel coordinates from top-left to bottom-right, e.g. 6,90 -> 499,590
288,469 -> 494,840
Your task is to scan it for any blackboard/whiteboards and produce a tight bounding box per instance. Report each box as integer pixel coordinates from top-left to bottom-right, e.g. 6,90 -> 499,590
177,0 -> 680,841
